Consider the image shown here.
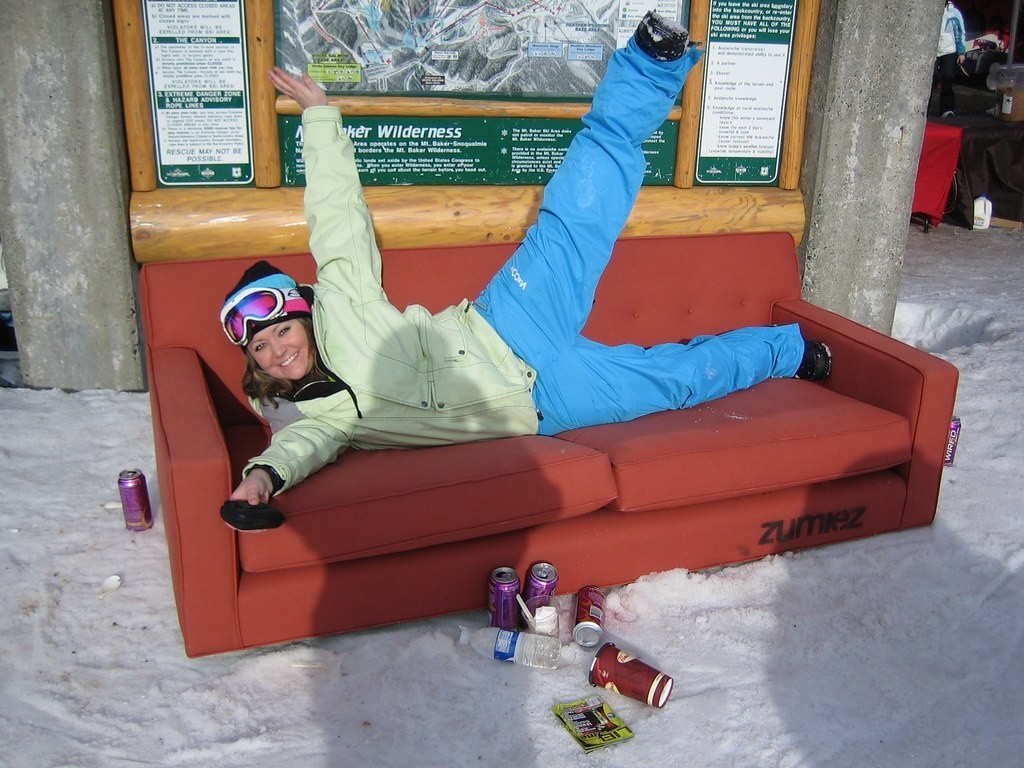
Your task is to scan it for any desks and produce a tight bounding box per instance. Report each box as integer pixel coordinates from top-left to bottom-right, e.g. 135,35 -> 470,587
912,115 -> 1024,233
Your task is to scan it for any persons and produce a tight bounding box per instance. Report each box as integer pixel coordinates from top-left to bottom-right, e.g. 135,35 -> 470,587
936,0 -> 1009,120
221,9 -> 834,532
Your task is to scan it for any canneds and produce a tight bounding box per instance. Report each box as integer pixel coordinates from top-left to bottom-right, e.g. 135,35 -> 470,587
943,416 -> 961,467
572,584 -> 606,647
118,468 -> 154,532
522,563 -> 559,629
488,567 -> 521,631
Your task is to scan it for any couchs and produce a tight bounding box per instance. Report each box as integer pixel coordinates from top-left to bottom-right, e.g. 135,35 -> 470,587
139,231 -> 959,658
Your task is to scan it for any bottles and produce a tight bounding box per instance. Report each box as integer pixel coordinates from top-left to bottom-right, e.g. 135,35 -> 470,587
995,63 -> 1024,123
459,627 -> 562,669
971,194 -> 992,230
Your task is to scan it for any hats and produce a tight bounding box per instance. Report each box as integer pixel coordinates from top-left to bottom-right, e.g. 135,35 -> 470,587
223,260 -> 311,344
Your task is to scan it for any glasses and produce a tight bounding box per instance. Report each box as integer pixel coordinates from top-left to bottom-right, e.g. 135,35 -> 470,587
219,288 -> 286,346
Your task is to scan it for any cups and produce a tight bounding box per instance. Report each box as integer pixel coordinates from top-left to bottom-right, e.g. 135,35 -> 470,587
521,596 -> 560,639
589,642 -> 673,709
555,593 -> 577,642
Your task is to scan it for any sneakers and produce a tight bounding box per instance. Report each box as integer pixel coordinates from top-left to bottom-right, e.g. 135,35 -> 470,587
635,10 -> 691,62
942,111 -> 954,118
794,340 -> 832,382
